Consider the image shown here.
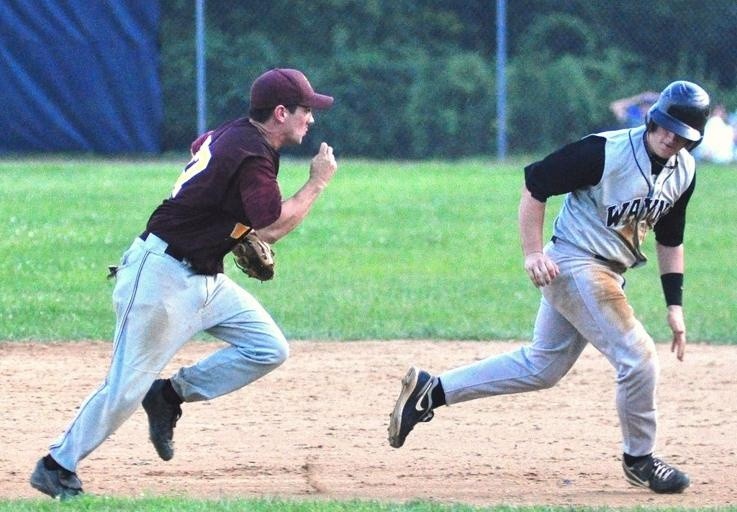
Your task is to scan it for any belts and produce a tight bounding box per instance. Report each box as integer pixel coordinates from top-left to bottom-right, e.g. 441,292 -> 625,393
551,235 -> 612,263
138,231 -> 184,262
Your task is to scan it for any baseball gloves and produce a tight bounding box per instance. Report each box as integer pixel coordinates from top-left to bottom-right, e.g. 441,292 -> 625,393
232,229 -> 275,281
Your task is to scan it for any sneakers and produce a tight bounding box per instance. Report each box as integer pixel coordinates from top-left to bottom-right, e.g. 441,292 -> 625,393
29,457 -> 85,501
140,377 -> 186,461
387,365 -> 439,448
622,454 -> 689,494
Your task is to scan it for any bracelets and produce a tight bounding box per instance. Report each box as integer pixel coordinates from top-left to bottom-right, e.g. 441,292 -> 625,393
658,271 -> 684,308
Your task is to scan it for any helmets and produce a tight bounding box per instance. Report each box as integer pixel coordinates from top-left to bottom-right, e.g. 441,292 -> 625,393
648,80 -> 713,143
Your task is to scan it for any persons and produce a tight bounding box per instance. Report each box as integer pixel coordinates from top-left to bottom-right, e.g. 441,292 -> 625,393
609,90 -> 736,165
29,67 -> 338,502
385,78 -> 712,495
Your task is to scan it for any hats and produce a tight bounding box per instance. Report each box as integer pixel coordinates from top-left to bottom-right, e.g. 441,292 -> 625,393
248,69 -> 334,113
